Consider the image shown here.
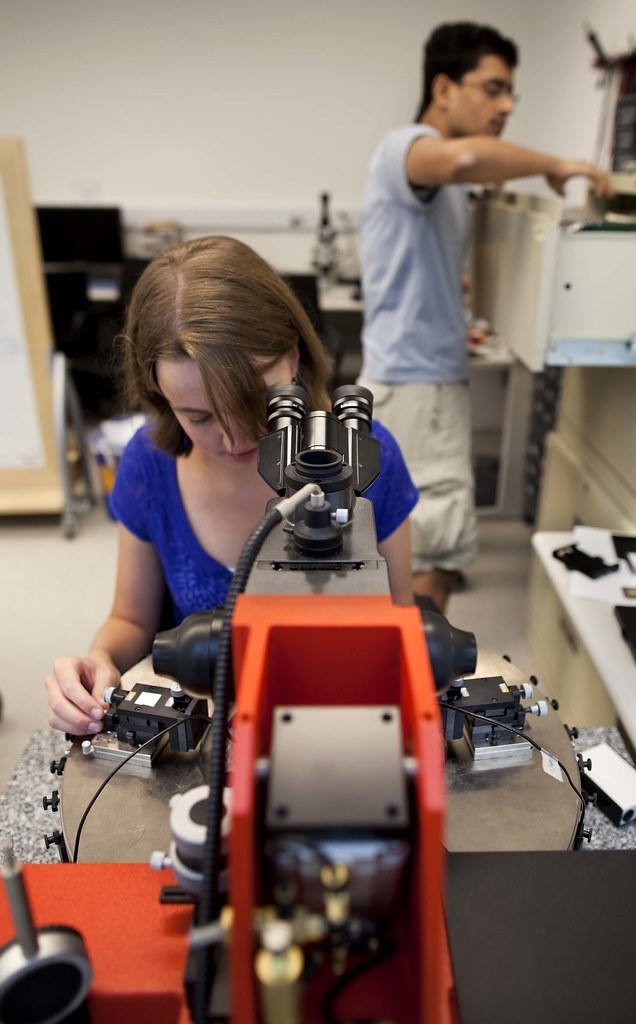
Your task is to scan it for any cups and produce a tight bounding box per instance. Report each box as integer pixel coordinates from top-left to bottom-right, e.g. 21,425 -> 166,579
143,221 -> 181,256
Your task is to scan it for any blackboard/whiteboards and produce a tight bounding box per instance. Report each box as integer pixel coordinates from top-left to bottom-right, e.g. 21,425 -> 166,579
598,61 -> 636,173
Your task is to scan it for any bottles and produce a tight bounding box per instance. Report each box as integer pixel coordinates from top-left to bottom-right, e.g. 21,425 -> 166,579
313,193 -> 338,273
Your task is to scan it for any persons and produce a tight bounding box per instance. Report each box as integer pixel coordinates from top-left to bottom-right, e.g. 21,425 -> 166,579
357,22 -> 616,616
44,236 -> 420,736
